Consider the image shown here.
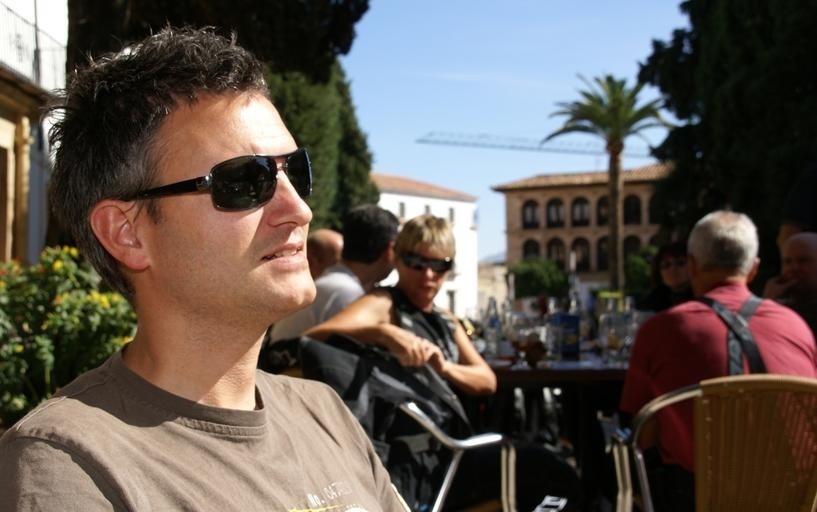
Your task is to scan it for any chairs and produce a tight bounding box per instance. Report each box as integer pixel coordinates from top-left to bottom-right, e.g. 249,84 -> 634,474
301,338 -> 517,512
612,376 -> 817,512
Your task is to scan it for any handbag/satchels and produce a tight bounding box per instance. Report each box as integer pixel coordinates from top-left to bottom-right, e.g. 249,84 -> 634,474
259,332 -> 478,512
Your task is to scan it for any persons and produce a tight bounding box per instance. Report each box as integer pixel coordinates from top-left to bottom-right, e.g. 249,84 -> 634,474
623,210 -> 817,512
299,213 -> 585,511
760,235 -> 815,335
303,205 -> 400,335
1,31 -> 411,512
638,233 -> 694,310
271,230 -> 345,341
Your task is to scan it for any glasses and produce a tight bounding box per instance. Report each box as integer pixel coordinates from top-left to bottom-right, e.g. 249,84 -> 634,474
123,148 -> 313,211
398,251 -> 455,273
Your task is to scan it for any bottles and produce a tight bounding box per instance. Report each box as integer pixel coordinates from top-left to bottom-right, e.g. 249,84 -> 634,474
622,296 -> 645,358
579,286 -> 598,349
599,296 -> 630,363
542,296 -> 565,361
506,299 -> 540,355
483,296 -> 501,357
567,289 -> 579,360
